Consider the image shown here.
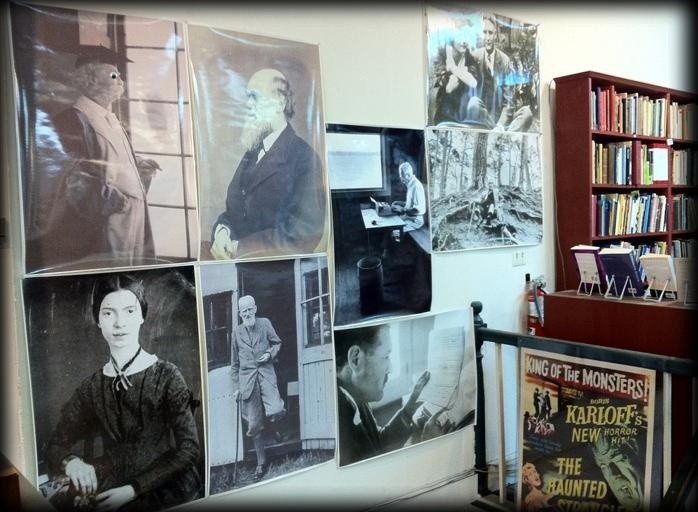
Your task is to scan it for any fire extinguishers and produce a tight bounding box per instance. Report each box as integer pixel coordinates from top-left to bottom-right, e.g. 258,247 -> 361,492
526,274 -> 549,337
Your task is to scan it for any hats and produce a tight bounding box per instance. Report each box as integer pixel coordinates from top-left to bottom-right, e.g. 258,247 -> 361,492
62,44 -> 137,68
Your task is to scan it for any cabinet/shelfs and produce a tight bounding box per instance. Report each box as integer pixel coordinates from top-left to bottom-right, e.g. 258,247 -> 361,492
544,289 -> 697,512
552,71 -> 698,296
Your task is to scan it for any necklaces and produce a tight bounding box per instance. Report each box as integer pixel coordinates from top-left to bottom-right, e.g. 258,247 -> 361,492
110,346 -> 142,401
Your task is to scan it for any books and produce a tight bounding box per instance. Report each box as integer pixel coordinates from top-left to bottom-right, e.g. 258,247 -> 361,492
571,80 -> 696,305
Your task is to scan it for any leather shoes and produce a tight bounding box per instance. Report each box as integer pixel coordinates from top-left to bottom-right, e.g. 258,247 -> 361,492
274,426 -> 287,444
253,466 -> 267,481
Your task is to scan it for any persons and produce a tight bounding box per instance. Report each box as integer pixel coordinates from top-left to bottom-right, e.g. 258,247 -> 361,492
45,274 -> 203,512
334,325 -> 457,465
209,69 -> 325,260
472,182 -> 505,227
381,162 -> 426,260
589,426 -> 644,512
434,16 -> 534,135
229,295 -> 288,479
524,386 -> 556,437
522,462 -> 560,511
38,43 -> 163,263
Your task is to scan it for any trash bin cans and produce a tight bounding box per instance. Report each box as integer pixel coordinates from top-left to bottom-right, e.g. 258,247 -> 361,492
357,256 -> 384,314
